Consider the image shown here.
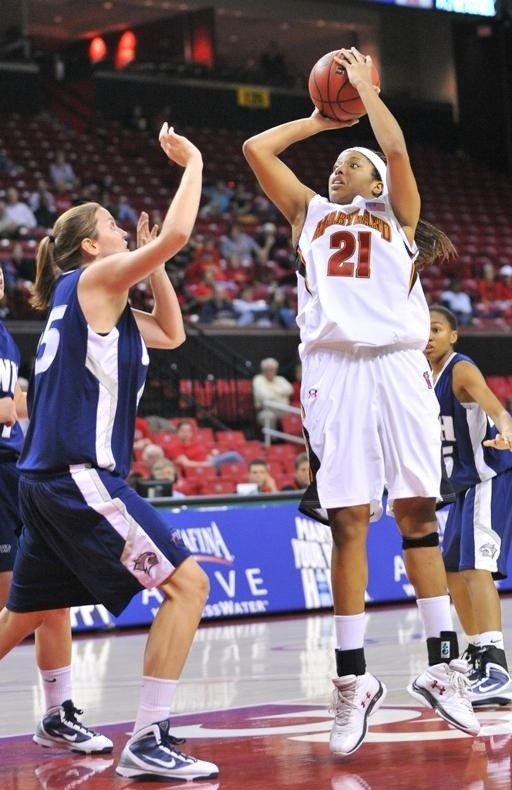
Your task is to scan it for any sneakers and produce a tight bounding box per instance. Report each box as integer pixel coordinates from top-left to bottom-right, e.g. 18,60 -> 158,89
112,721 -> 224,779
322,670 -> 389,758
30,698 -> 112,758
409,630 -> 512,741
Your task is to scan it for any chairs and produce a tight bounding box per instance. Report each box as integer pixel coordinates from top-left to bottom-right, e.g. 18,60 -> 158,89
1,95 -> 511,504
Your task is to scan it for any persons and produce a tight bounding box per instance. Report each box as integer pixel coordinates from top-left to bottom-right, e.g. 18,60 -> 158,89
244,45 -> 481,756
425,305 -> 512,705
0,123 -> 223,780
1,44 -> 512,502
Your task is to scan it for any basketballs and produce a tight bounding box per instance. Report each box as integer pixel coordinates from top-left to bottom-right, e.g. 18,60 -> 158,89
309,50 -> 379,121
